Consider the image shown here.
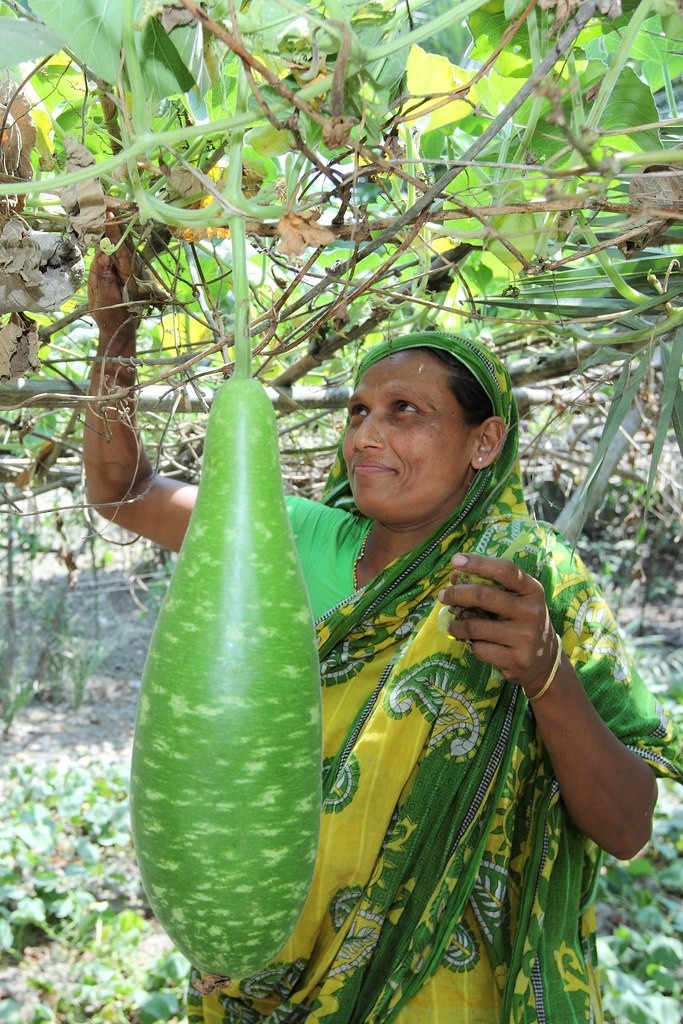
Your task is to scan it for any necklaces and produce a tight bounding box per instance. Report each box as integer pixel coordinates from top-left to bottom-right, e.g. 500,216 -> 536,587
352,523 -> 374,591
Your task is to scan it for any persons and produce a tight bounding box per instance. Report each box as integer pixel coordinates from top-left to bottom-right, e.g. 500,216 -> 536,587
80,208 -> 683,1024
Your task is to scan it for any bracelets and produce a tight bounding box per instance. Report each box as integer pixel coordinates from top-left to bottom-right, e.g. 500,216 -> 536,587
520,630 -> 564,702
